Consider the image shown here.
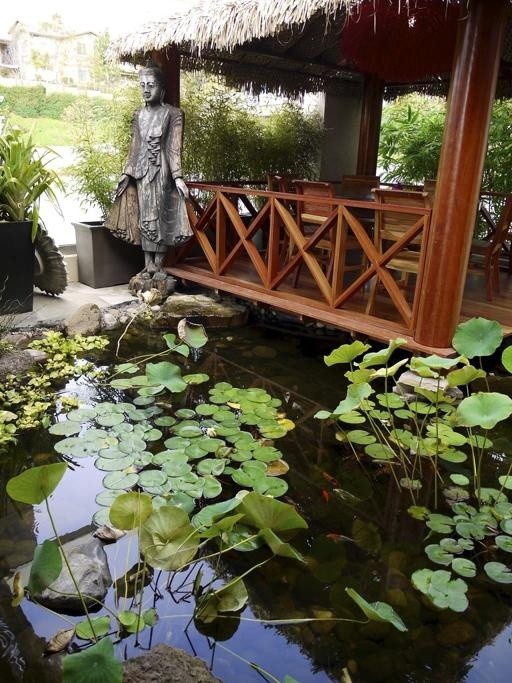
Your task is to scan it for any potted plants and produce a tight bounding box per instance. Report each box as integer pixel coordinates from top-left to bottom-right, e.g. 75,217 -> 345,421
0,111 -> 65,318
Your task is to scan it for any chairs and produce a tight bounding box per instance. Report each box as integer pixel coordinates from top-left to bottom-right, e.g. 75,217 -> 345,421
259,172 -> 512,314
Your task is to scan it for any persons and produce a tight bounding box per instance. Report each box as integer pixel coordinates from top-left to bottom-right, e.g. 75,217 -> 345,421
113,65 -> 193,280
